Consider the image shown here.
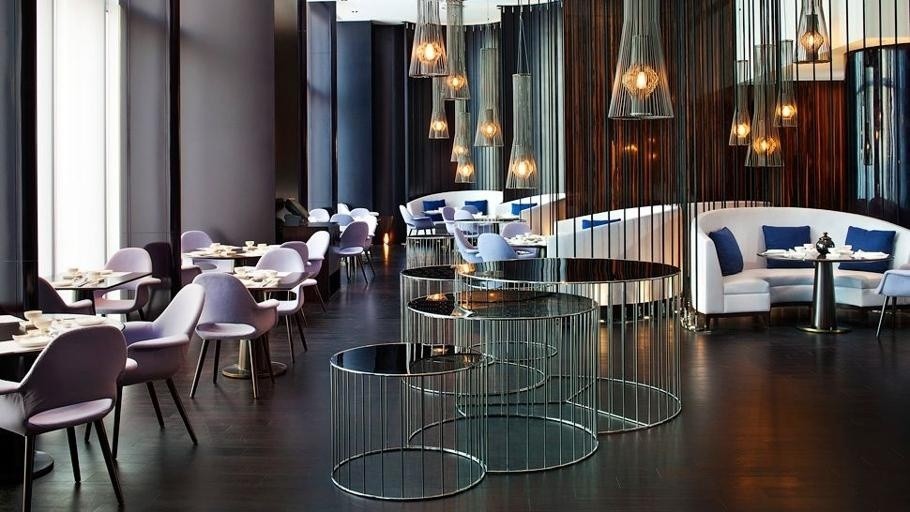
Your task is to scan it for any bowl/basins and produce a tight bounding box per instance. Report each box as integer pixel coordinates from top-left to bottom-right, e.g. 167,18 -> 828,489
245,241 -> 254,247
254,270 -> 278,279
34,319 -> 53,331
224,246 -> 232,252
63,275 -> 74,280
234,267 -> 245,275
24,310 -> 42,322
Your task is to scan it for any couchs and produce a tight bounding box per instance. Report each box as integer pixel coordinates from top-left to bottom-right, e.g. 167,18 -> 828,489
691,207 -> 910,336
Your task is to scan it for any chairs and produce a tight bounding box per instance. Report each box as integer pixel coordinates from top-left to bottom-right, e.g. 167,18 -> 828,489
399,203 -> 541,261
546,204 -> 684,324
497,193 -> 565,237
871,269 -> 910,338
0,203 -> 378,511
406,190 -> 503,237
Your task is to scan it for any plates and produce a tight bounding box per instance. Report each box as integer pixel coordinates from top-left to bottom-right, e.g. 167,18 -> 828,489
69,268 -> 112,283
56,281 -> 73,286
75,315 -> 107,325
12,334 -> 52,346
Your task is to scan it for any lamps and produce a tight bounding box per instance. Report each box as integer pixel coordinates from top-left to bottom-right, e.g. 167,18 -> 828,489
408,0 -> 832,190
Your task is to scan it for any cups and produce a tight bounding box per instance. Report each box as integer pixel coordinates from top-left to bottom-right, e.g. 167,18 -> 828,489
511,233 -> 532,245
827,243 -> 865,257
785,243 -> 817,259
258,244 -> 266,252
210,242 -> 220,249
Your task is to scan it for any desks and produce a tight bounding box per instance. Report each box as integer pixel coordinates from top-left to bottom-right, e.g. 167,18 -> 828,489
327,339 -> 487,500
399,264 -> 551,397
457,258 -> 687,438
407,288 -> 600,479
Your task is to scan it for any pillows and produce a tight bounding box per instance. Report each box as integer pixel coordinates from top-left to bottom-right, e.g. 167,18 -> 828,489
422,199 -> 447,221
581,218 -> 621,229
762,224 -> 814,268
708,226 -> 744,276
838,225 -> 896,273
464,199 -> 488,216
